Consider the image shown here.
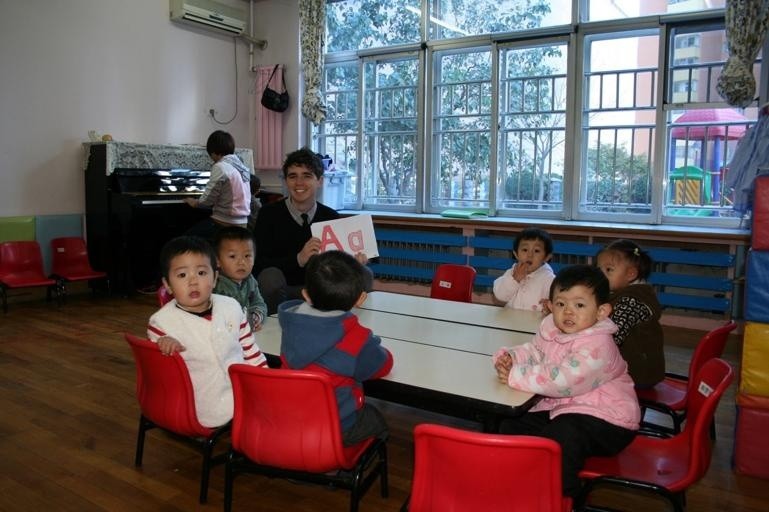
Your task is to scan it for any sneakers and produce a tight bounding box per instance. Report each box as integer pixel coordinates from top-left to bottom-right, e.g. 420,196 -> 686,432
137,282 -> 162,296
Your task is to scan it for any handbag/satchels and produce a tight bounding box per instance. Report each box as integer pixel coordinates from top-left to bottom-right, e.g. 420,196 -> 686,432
261,64 -> 289,112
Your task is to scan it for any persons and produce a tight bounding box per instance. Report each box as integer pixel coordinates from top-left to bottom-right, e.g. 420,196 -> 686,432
246,171 -> 263,229
280,250 -> 396,491
142,233 -> 280,433
206,226 -> 269,333
492,228 -> 560,310
540,239 -> 669,390
487,263 -> 643,512
135,129 -> 252,295
249,143 -> 374,314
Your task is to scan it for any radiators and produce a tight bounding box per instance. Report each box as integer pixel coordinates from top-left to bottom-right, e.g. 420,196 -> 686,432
253,63 -> 284,171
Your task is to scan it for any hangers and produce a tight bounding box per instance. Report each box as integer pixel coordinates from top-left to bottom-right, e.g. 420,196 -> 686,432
248,64 -> 274,95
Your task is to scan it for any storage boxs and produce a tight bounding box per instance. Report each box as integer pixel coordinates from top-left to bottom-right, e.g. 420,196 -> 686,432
279,167 -> 349,211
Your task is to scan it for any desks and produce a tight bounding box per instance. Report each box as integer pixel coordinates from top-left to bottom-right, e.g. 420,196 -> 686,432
245,289 -> 600,459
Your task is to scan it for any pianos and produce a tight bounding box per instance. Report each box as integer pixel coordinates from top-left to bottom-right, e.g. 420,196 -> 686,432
85,141 -> 255,271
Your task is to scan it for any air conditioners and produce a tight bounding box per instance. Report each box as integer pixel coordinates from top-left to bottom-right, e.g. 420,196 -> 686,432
170,1 -> 246,38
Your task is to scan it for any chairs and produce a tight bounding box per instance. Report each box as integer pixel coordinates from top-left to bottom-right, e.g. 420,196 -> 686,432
616,318 -> 737,443
123,330 -> 261,505
0,240 -> 55,310
157,284 -> 179,310
50,237 -> 110,301
430,264 -> 478,304
402,421 -> 575,511
222,359 -> 393,511
572,355 -> 735,511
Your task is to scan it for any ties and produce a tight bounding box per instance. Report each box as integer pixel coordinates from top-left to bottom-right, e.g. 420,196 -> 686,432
301,213 -> 309,226
